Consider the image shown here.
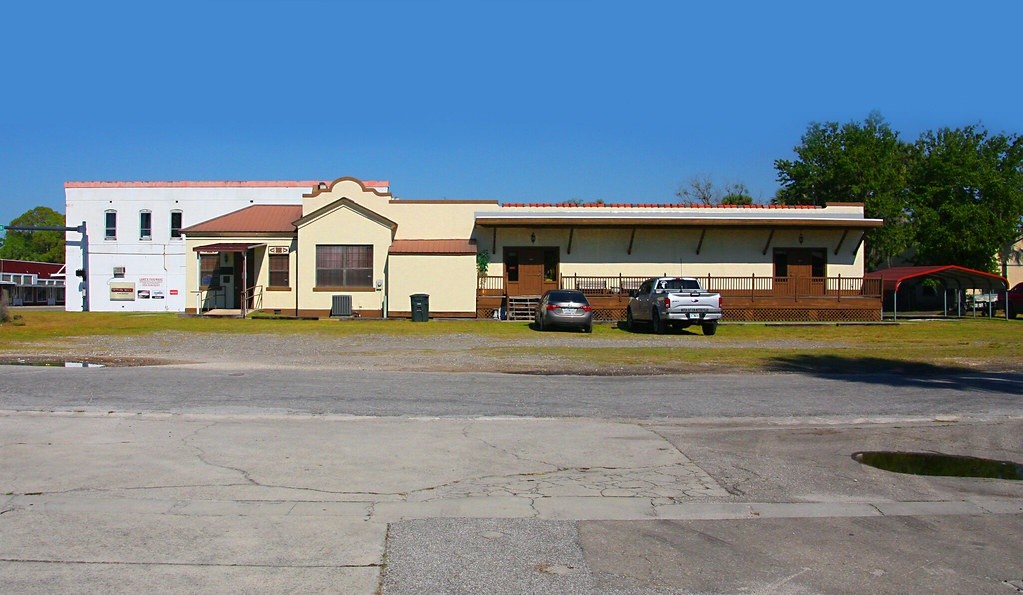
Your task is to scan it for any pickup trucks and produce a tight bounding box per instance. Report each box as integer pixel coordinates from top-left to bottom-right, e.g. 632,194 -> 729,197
626,276 -> 724,335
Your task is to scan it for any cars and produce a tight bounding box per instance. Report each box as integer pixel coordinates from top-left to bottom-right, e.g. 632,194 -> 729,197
998,282 -> 1023,320
532,288 -> 593,333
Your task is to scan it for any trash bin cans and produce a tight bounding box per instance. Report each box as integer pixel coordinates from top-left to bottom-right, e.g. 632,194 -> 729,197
409,294 -> 430,322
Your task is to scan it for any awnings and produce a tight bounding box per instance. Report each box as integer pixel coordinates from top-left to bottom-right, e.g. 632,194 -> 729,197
193,243 -> 267,314
473,211 -> 885,255
